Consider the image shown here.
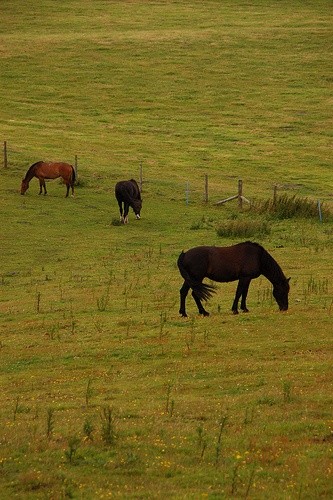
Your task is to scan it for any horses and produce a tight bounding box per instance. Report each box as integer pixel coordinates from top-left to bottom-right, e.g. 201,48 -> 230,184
19,161 -> 76,198
114,178 -> 143,224
176,241 -> 292,317
